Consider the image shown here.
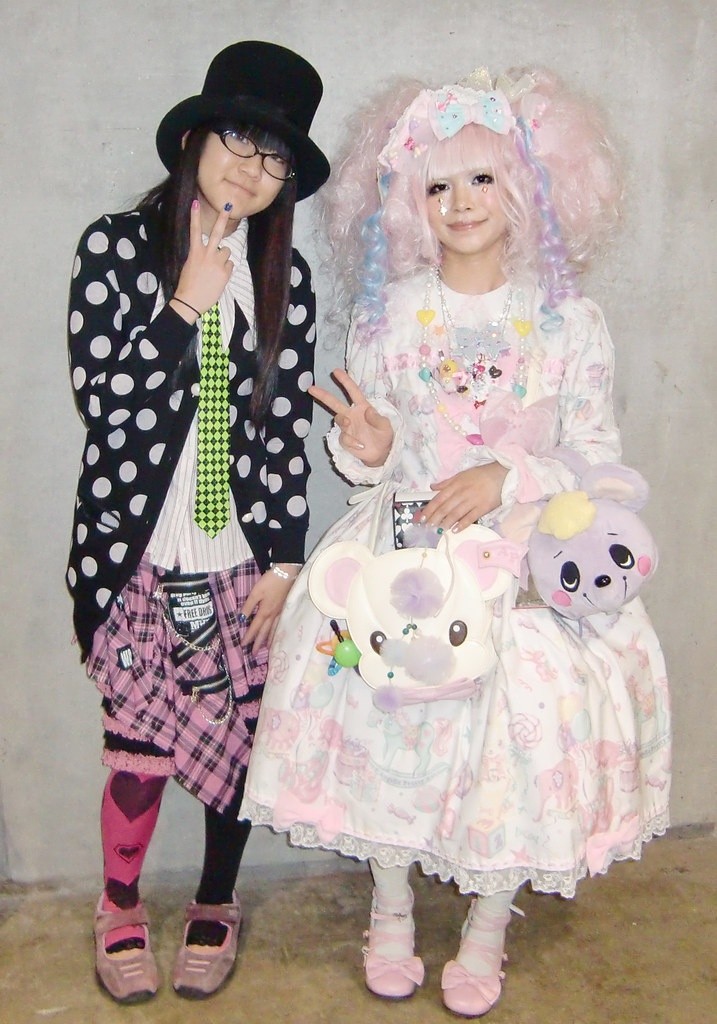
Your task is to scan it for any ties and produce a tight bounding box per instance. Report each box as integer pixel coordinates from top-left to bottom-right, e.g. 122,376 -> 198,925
195,301 -> 230,540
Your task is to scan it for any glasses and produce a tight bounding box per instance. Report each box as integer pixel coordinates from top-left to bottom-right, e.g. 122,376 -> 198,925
207,123 -> 296,181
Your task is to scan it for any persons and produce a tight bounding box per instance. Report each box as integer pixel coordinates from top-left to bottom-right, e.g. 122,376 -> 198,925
238,67 -> 671,1015
67,41 -> 332,1006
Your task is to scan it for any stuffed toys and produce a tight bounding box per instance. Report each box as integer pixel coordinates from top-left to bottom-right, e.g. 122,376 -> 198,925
500,462 -> 658,621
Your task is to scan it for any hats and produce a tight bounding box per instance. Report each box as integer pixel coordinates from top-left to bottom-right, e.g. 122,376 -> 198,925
155,40 -> 331,201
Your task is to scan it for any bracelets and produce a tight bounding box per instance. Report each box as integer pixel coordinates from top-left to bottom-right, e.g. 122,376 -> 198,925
171,297 -> 202,317
272,566 -> 299,580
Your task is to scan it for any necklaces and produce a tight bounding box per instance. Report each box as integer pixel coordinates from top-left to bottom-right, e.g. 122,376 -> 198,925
418,259 -> 530,446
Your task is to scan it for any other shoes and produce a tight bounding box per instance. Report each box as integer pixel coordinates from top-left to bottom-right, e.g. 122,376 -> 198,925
360,883 -> 424,998
94,885 -> 157,1004
442,896 -> 512,1015
172,887 -> 243,1000
